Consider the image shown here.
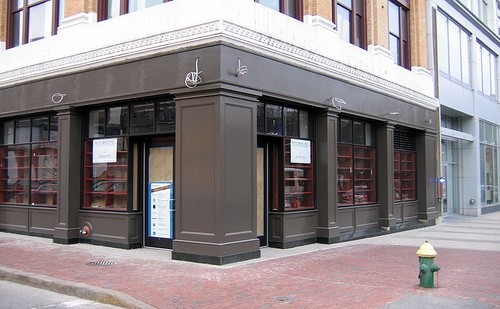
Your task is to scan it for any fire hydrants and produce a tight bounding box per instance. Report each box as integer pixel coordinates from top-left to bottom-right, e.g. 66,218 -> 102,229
417,240 -> 440,289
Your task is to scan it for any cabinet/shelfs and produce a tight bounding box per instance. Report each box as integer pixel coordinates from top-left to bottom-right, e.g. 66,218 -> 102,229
272,138 -> 314,213
83,137 -> 128,211
0,144 -> 29,205
31,142 -> 59,207
394,151 -> 401,201
401,151 -> 416,200
354,146 -> 377,204
336,144 -> 353,206
